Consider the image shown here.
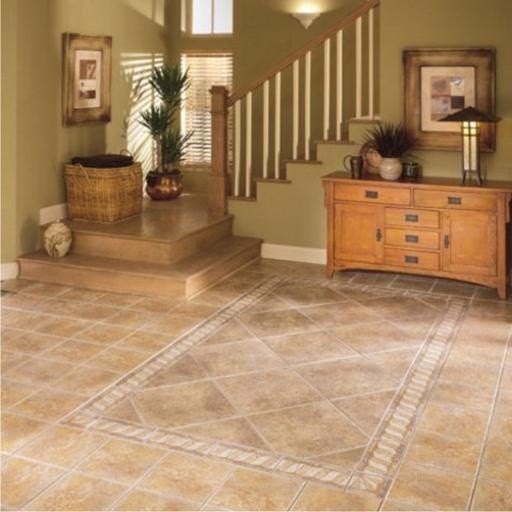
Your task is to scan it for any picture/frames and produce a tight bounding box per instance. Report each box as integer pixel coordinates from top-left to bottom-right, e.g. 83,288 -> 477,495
59,30 -> 113,125
402,48 -> 497,153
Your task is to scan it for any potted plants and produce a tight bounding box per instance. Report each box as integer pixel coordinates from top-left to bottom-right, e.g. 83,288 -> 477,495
358,119 -> 423,181
135,61 -> 196,202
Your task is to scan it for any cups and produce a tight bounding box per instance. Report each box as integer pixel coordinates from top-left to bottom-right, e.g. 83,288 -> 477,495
402,161 -> 421,180
350,154 -> 363,179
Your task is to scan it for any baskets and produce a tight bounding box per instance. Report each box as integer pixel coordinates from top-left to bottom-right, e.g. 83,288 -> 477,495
62,149 -> 145,224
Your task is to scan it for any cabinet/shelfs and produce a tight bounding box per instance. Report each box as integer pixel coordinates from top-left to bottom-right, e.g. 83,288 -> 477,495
320,170 -> 512,301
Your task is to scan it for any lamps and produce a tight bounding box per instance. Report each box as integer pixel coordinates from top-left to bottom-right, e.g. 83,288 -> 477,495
291,11 -> 322,30
435,107 -> 503,187
